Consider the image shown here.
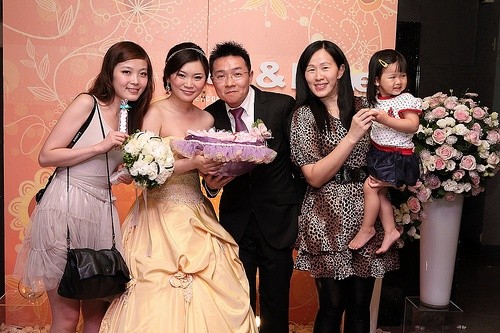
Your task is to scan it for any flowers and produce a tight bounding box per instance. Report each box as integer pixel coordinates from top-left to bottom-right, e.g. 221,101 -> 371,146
250,119 -> 275,148
108,130 -> 175,190
392,88 -> 500,248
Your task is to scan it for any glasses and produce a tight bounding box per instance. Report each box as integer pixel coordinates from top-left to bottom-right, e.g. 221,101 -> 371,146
211,69 -> 250,79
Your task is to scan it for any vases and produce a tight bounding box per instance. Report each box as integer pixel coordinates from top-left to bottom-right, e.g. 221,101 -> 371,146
418,191 -> 464,308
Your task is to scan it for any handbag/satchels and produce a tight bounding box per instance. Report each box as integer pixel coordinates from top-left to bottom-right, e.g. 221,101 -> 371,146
57,246 -> 131,300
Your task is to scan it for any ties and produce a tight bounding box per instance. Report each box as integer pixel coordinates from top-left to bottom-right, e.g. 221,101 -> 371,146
229,107 -> 250,132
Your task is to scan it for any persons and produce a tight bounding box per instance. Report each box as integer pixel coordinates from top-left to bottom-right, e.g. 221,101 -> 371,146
290,40 -> 402,333
24,41 -> 154,332
350,49 -> 422,255
202,39 -> 299,333
137,42 -> 226,333
